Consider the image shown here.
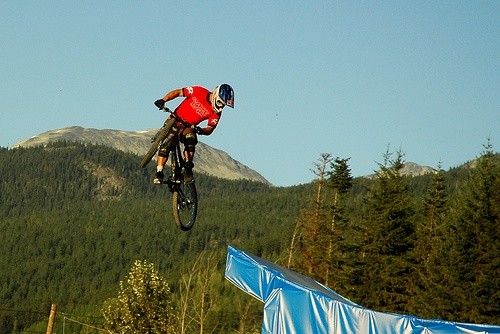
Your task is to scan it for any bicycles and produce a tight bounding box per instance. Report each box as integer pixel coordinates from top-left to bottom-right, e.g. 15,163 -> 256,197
141,104 -> 200,231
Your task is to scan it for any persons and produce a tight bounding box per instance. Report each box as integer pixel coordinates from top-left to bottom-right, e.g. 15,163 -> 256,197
153,83 -> 234,184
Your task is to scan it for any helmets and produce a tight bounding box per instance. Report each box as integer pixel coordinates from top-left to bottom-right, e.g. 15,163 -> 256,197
212,83 -> 234,113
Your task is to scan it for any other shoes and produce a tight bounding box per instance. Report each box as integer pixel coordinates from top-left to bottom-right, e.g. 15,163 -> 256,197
154,171 -> 165,184
181,167 -> 195,184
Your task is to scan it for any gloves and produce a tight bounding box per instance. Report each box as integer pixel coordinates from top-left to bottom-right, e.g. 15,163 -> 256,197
154,99 -> 166,111
194,126 -> 204,135
